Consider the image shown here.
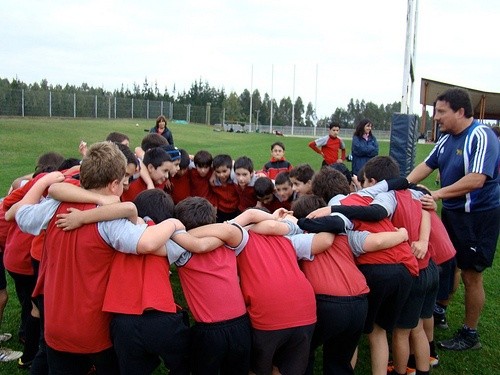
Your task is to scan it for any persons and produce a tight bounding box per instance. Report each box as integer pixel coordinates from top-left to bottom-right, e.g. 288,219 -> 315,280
308,123 -> 345,168
351,119 -> 379,179
405,88 -> 500,350
0,133 -> 456,375
149,114 -> 174,149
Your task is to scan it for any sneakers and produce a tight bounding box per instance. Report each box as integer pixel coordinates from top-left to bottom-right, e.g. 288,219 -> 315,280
18,357 -> 24,366
437,327 -> 481,350
0,332 -> 12,343
429,350 -> 439,367
0,349 -> 23,362
435,315 -> 450,330
387,353 -> 416,375
